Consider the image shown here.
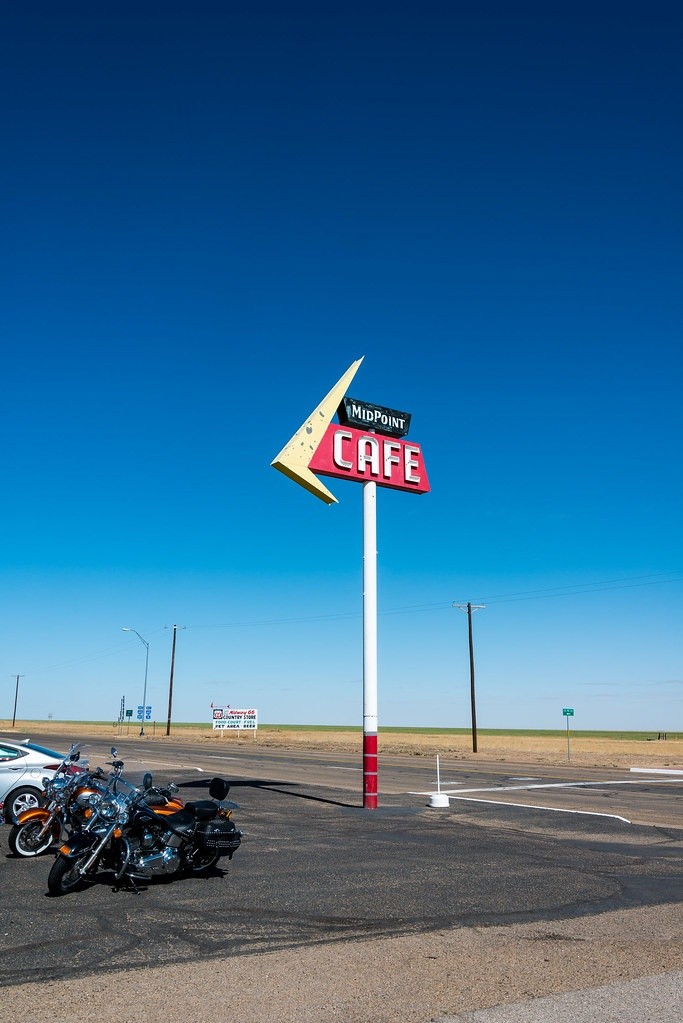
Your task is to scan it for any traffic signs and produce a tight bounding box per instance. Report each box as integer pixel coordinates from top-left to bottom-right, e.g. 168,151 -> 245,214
146,710 -> 151,714
138,710 -> 144,715
126,710 -> 132,716
146,715 -> 151,719
146,706 -> 152,709
138,706 -> 144,709
563,709 -> 575,716
137,715 -> 142,719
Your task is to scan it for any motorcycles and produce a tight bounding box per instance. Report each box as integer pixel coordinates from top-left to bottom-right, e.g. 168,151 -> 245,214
47,748 -> 243,896
8,743 -> 184,858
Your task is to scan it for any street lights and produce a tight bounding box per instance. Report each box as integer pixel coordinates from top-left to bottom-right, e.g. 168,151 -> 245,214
123,628 -> 150,736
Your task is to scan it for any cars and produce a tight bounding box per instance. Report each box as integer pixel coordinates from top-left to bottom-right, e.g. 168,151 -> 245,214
0,737 -> 90,825
